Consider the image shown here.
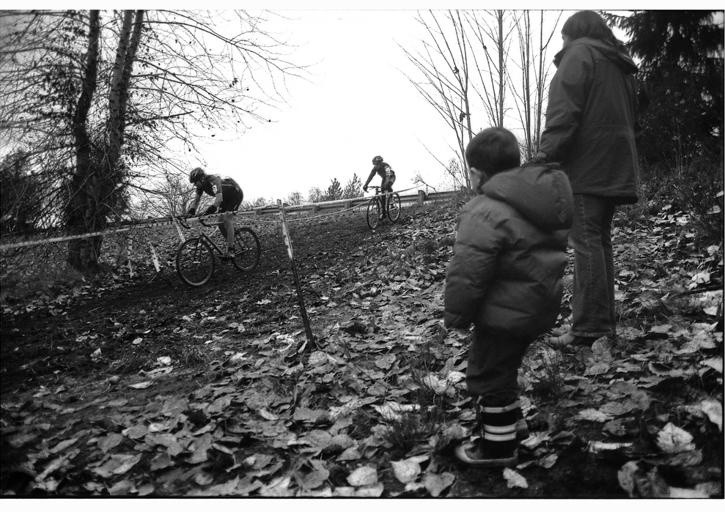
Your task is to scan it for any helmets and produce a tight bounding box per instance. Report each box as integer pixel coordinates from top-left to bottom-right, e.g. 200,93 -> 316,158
371,156 -> 384,165
189,167 -> 204,185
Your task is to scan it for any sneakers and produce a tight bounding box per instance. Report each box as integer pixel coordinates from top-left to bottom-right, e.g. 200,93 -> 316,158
388,197 -> 395,205
228,244 -> 237,258
454,436 -> 518,467
470,422 -> 531,442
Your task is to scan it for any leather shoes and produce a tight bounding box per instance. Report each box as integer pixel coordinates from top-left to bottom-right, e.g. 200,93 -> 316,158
547,329 -> 596,354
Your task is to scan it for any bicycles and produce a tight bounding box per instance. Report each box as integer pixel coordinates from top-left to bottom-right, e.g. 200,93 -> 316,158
176,212 -> 262,288
364,185 -> 402,230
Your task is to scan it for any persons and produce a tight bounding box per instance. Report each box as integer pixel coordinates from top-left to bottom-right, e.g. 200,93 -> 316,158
443,126 -> 576,465
182,166 -> 243,260
362,155 -> 397,221
529,10 -> 643,353
